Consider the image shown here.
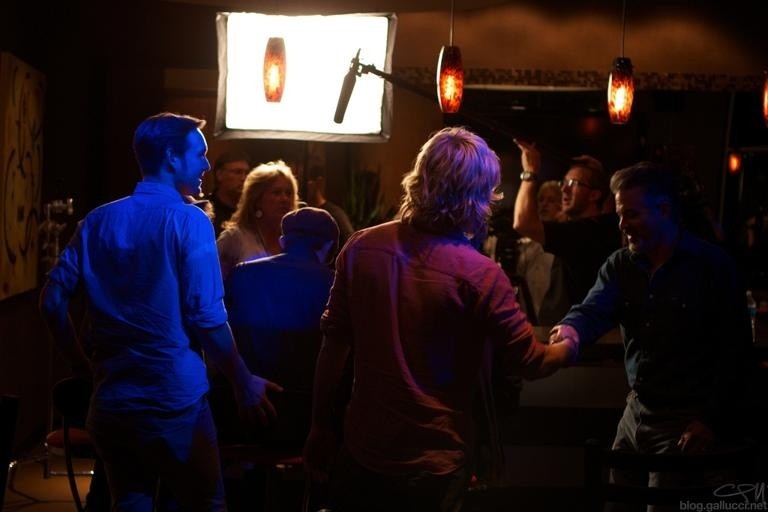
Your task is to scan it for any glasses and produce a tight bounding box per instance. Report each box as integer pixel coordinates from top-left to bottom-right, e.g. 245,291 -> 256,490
217,164 -> 253,176
556,176 -> 597,194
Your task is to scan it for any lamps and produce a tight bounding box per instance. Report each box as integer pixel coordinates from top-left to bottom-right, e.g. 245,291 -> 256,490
434,0 -> 465,117
604,1 -> 635,130
262,32 -> 288,104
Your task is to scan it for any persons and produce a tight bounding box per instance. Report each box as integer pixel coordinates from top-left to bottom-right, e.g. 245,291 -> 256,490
42,112 -> 728,512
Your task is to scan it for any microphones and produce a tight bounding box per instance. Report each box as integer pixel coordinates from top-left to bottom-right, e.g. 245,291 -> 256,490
333,59 -> 359,123
